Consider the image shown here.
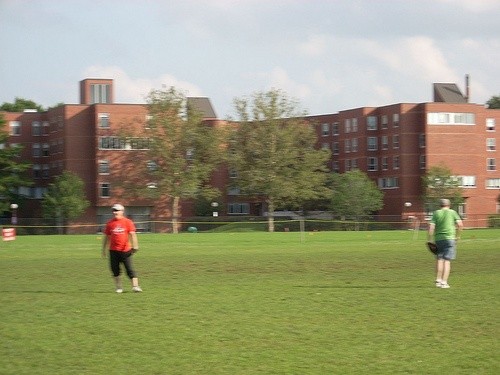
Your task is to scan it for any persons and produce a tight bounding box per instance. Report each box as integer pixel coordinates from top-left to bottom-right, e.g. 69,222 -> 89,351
427,198 -> 464,288
102,203 -> 142,294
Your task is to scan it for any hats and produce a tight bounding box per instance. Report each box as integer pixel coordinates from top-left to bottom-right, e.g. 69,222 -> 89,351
111,204 -> 124,211
439,199 -> 451,206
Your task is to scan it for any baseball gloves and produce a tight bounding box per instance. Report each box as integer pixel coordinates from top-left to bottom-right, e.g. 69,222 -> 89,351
426,242 -> 438,255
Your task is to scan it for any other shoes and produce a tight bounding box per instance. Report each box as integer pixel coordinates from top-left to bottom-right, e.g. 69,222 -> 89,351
116,288 -> 123,293
133,286 -> 142,292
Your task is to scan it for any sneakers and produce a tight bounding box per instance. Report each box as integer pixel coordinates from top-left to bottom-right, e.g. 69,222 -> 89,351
434,280 -> 442,286
440,282 -> 450,288
